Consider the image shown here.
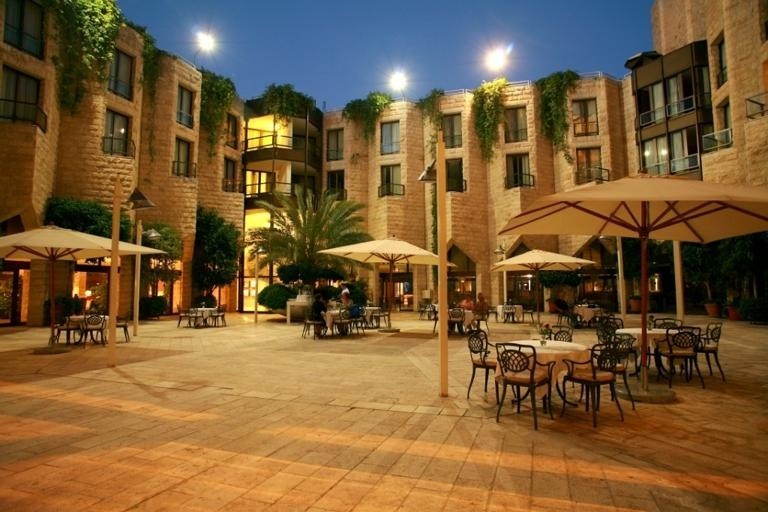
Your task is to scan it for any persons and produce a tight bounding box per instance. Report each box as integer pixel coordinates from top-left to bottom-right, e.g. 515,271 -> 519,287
311,272 -> 365,336
462,291 -> 487,332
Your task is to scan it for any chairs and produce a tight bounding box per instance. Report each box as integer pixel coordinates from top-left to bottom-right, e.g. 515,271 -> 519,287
177,302 -> 230,332
466,299 -> 726,430
300,297 -> 492,344
47,293 -> 133,350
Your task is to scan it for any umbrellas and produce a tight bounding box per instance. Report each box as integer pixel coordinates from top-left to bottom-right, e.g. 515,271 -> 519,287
489,249 -> 597,329
496,168 -> 768,392
0,223 -> 169,344
316,236 -> 458,328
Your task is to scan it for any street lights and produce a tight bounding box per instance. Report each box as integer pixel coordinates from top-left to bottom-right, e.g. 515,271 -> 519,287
250,234 -> 268,324
416,128 -> 455,400
493,244 -> 507,305
130,216 -> 162,338
103,171 -> 155,344
598,233 -> 628,319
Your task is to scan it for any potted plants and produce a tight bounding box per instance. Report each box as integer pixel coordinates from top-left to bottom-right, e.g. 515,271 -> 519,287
660,234 -> 761,324
135,224 -> 183,318
194,204 -> 241,308
